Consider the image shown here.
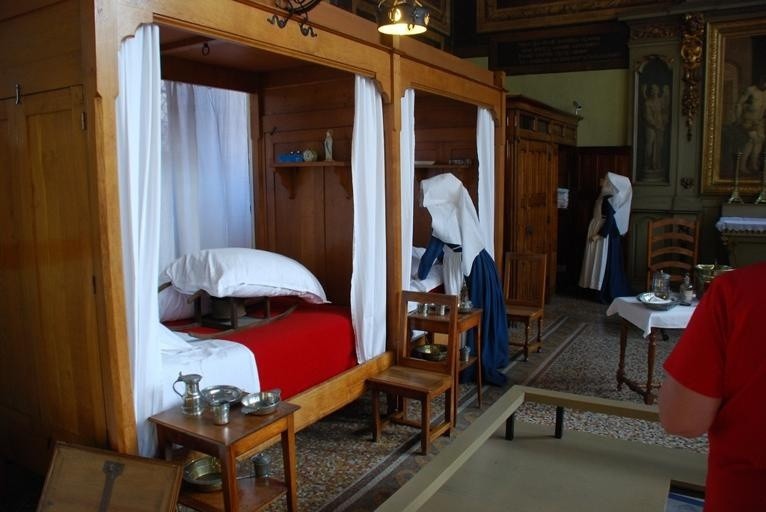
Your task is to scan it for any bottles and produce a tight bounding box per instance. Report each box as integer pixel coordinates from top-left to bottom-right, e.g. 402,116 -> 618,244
172,373 -> 206,416
653,271 -> 694,306
462,282 -> 469,302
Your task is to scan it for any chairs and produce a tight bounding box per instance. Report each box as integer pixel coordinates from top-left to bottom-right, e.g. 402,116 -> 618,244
503,252 -> 548,362
365,290 -> 458,455
647,213 -> 701,338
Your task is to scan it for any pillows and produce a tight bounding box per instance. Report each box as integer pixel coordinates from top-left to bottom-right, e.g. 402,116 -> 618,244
165,247 -> 332,306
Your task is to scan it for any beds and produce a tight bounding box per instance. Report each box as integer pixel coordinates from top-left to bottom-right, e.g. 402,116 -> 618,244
1,0 -> 508,475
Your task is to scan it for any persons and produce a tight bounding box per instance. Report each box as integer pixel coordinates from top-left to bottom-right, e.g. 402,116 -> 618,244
656,262 -> 766,511
735,77 -> 766,176
642,79 -> 667,170
413,173 -> 509,386
580,172 -> 633,303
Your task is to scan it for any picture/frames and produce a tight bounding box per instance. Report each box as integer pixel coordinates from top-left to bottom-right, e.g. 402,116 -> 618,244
700,17 -> 766,196
633,54 -> 674,186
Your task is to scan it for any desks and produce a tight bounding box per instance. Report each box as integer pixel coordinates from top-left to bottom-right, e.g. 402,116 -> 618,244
147,391 -> 302,512
407,308 -> 484,428
606,295 -> 700,404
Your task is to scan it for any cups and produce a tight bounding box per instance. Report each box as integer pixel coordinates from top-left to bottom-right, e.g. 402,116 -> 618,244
208,400 -> 231,426
460,346 -> 471,362
251,453 -> 272,478
417,303 -> 449,316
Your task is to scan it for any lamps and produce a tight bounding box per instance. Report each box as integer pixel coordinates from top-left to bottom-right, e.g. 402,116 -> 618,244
268,0 -> 431,38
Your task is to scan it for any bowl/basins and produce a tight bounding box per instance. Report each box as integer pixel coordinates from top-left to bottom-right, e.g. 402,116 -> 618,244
414,344 -> 448,361
695,263 -> 736,299
181,455 -> 242,492
636,292 -> 680,311
458,303 -> 473,313
239,389 -> 282,416
201,385 -> 242,407
